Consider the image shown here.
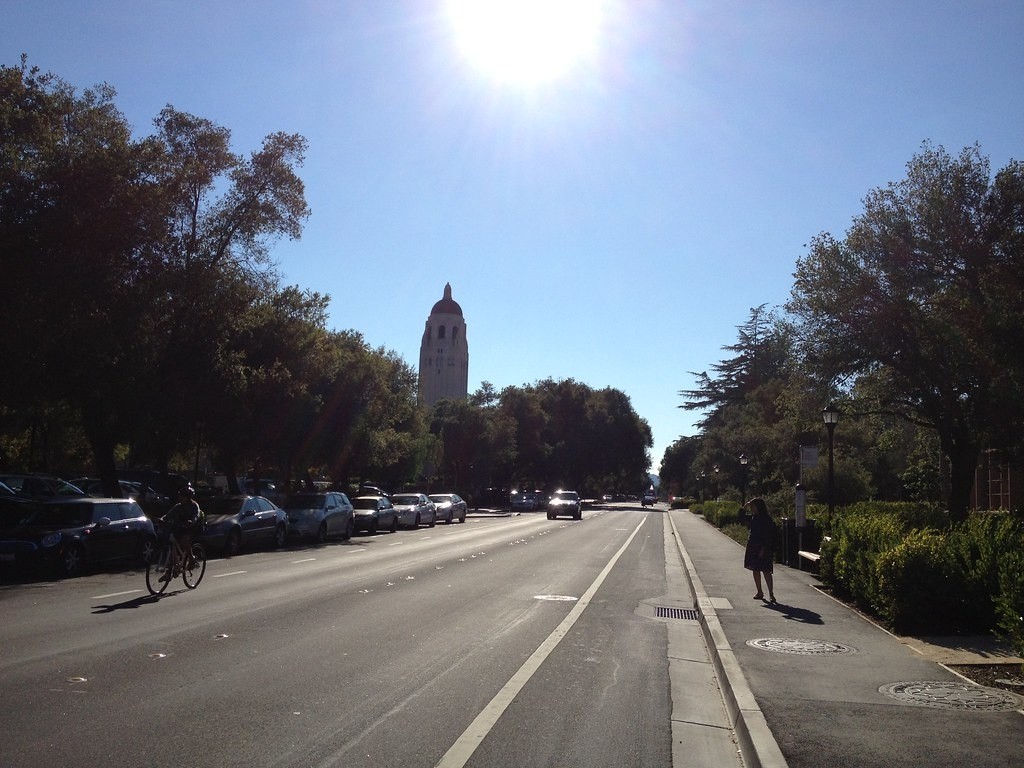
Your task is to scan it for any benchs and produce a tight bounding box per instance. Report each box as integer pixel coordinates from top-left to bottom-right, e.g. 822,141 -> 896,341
797,536 -> 831,575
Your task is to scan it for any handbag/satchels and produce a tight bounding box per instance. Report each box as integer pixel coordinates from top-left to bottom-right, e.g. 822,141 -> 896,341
196,511 -> 208,535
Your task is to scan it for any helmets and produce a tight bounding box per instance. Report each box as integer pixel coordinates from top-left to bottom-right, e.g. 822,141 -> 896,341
179,487 -> 196,496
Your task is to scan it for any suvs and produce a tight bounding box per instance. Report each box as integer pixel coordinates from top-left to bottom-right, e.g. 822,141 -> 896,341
547,490 -> 581,520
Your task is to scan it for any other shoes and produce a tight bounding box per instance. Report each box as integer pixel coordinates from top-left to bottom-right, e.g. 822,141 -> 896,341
159,572 -> 172,582
754,592 -> 763,599
187,556 -> 197,570
770,595 -> 775,602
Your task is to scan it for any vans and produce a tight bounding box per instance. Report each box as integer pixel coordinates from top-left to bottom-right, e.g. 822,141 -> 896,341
645,496 -> 653,506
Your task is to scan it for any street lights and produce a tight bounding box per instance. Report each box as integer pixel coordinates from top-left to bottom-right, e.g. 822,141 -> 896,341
739,453 -> 748,507
701,471 -> 705,504
714,465 -> 720,501
696,475 -> 700,503
821,401 -> 841,537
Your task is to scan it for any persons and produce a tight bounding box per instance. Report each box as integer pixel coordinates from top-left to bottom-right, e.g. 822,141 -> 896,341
738,498 -> 778,602
156,487 -> 200,581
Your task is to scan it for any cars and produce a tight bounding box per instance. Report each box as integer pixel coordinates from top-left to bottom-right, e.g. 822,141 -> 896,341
603,494 -> 637,500
390,493 -> 437,530
0,498 -> 158,581
428,494 -> 467,524
197,495 -> 290,556
513,493 -> 538,511
472,488 -> 545,505
282,492 -> 355,544
349,496 -> 398,536
0,468 -> 388,504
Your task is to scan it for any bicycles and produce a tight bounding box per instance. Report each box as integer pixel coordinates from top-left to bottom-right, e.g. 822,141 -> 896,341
146,519 -> 206,595
